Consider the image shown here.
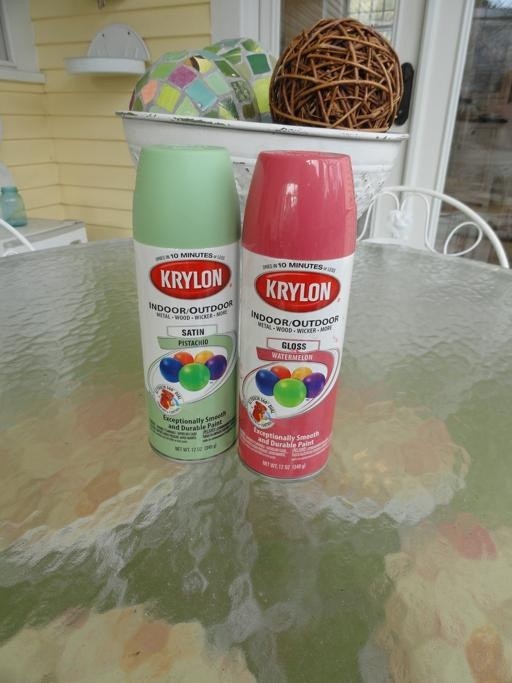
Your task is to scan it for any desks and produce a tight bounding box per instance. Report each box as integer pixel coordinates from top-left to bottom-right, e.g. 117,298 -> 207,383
0,238 -> 510,683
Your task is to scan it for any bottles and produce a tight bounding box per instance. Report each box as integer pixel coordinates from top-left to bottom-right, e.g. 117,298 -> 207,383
1,186 -> 29,227
241,148 -> 361,478
136,143 -> 240,457
